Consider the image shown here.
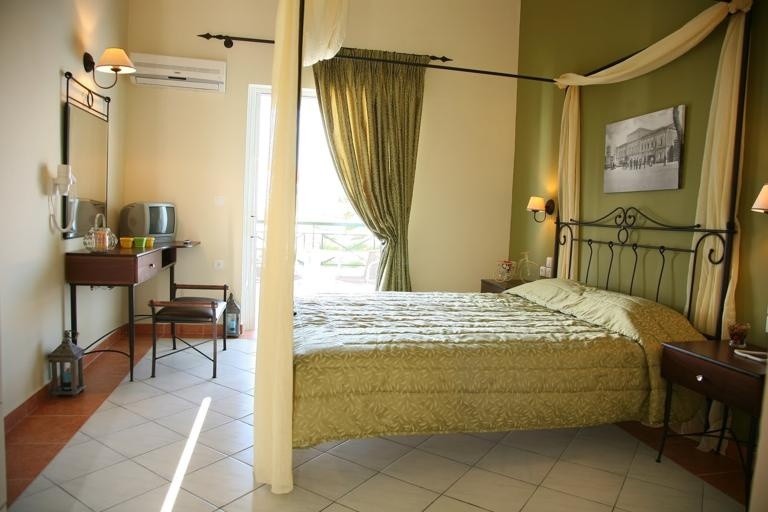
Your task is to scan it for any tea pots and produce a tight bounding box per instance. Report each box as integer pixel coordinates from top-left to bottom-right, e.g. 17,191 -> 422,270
83,213 -> 118,253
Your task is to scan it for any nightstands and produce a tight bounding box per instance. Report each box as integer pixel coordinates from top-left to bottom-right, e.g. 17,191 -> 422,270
656,338 -> 768,511
481,277 -> 533,293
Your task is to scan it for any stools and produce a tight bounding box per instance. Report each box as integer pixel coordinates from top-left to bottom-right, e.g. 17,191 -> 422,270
148,283 -> 230,377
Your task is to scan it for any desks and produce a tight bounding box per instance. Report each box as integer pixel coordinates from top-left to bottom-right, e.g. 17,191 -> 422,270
64,238 -> 201,382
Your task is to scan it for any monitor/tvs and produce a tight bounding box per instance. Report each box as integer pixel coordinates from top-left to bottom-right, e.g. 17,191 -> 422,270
120,202 -> 178,243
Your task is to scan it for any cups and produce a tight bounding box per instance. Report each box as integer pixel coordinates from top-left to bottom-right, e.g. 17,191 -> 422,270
727,320 -> 752,349
120,237 -> 155,248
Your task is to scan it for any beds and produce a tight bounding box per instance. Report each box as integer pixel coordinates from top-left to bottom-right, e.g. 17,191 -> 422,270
292,205 -> 738,450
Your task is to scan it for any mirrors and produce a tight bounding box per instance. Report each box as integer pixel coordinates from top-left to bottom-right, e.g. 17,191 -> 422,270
62,70 -> 111,240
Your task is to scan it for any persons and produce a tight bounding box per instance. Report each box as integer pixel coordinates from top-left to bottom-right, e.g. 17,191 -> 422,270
626,153 -> 670,169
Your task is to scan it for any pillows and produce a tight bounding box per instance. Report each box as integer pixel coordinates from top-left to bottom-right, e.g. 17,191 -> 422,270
503,276 -> 710,437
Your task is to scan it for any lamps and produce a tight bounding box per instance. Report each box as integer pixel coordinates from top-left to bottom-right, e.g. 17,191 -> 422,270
750,183 -> 768,215
83,47 -> 137,89
46,330 -> 87,397
43,162 -> 72,197
225,292 -> 240,338
526,196 -> 555,223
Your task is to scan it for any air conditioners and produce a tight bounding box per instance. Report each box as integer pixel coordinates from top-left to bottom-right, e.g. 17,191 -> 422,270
130,51 -> 227,92
